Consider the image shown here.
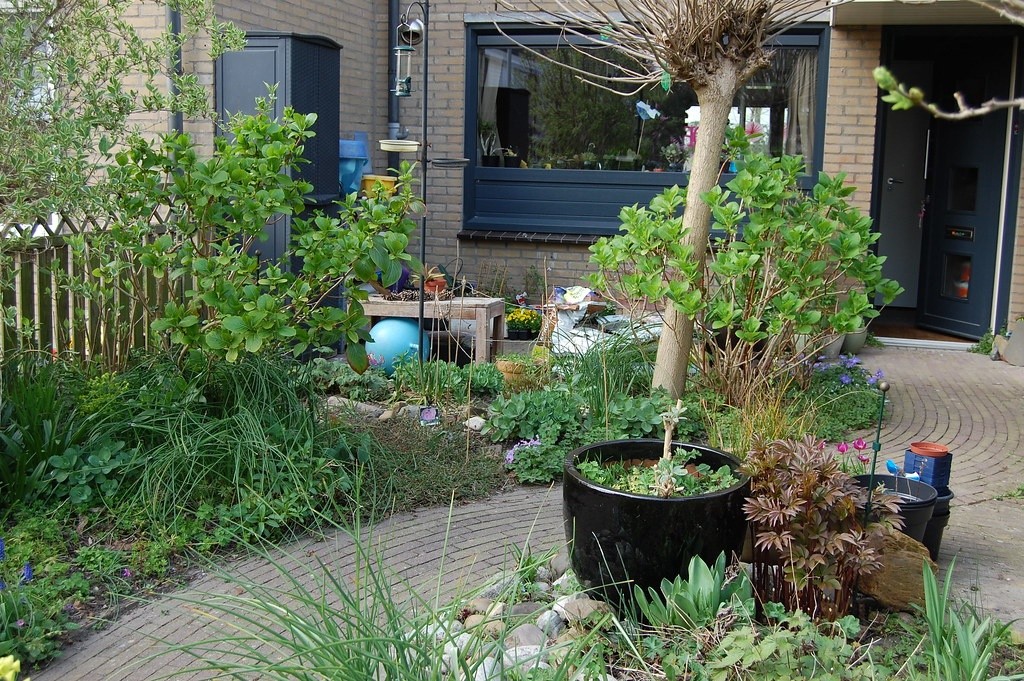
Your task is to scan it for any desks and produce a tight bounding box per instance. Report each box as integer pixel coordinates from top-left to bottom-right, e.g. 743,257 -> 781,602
347,294 -> 506,371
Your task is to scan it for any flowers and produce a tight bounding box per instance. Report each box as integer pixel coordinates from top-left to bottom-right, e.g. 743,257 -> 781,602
506,308 -> 543,330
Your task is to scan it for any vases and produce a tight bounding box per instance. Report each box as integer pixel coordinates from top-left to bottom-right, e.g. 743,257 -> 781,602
842,442 -> 954,561
821,335 -> 845,357
790,334 -> 819,355
844,328 -> 868,355
508,330 -> 538,340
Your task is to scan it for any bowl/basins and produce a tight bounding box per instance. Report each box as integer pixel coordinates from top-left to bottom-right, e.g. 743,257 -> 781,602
340,139 -> 369,157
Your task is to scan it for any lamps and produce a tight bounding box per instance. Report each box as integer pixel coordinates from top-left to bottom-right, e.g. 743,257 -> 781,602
400,16 -> 424,45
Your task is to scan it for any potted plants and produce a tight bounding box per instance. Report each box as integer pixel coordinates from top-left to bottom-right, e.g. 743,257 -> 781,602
504,147 -> 521,167
534,126 -> 762,174
410,263 -> 448,296
498,353 -> 545,393
479,121 -> 504,166
563,400 -> 753,632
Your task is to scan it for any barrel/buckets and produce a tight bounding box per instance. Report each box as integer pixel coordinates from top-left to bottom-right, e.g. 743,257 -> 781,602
842,474 -> 938,549
339,157 -> 369,194
922,483 -> 957,561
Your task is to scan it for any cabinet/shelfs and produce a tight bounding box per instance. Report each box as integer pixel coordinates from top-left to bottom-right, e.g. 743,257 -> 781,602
216,28 -> 346,363
